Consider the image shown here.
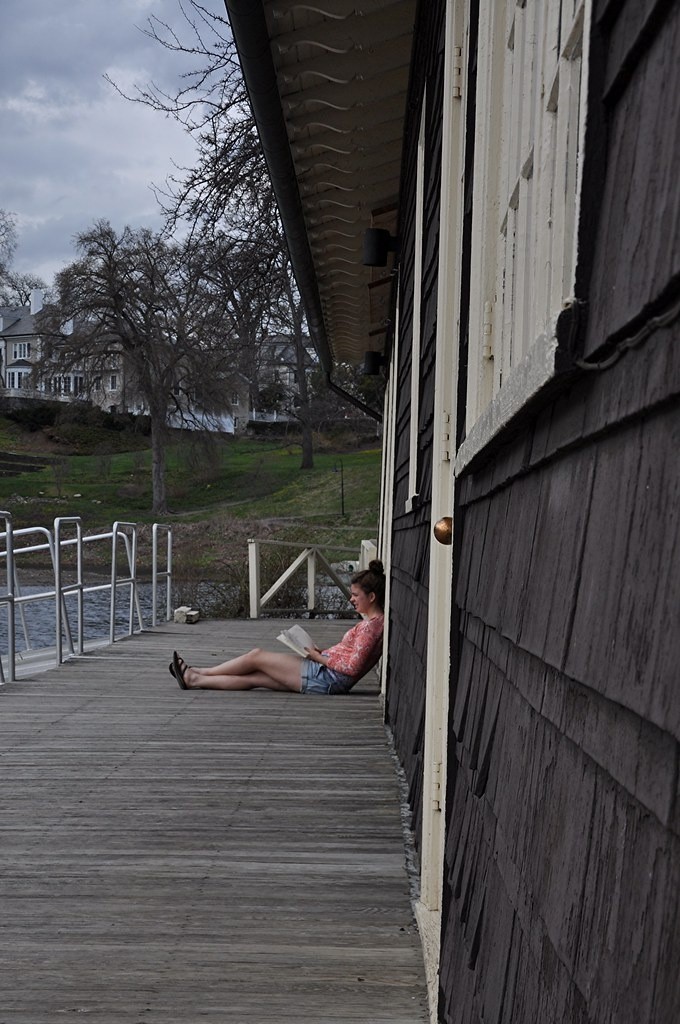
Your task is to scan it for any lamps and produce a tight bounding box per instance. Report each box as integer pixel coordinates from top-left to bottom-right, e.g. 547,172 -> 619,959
330,459 -> 342,473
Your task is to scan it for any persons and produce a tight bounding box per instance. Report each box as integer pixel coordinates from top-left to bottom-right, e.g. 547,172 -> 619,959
169,560 -> 386,694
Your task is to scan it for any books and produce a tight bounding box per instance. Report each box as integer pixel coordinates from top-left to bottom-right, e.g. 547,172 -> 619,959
277,625 -> 314,658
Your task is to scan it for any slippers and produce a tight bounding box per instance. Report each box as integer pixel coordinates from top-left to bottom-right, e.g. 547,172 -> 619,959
169,663 -> 191,679
173,651 -> 188,690
